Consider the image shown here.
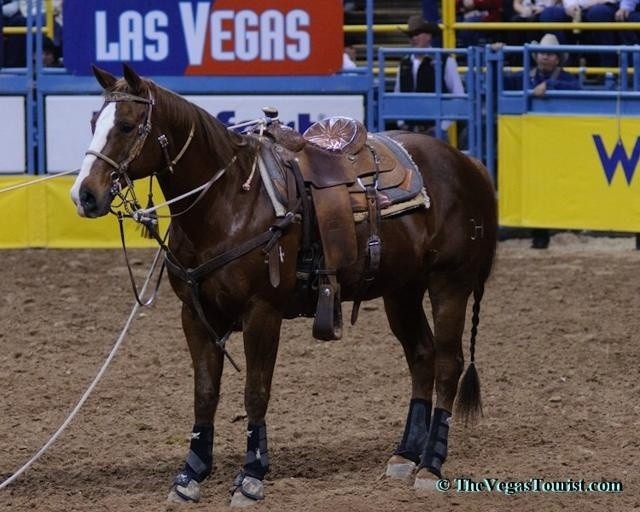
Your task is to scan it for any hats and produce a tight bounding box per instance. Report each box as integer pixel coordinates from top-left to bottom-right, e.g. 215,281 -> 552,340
531,34 -> 569,65
398,15 -> 442,39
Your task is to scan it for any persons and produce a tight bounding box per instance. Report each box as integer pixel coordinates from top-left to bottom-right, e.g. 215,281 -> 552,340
436,1 -> 640,68
492,33 -> 584,98
343,39 -> 359,71
393,16 -> 465,144
6,1 -> 63,67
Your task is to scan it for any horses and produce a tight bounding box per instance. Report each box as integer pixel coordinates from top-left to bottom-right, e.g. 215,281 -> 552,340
69,63 -> 501,509
